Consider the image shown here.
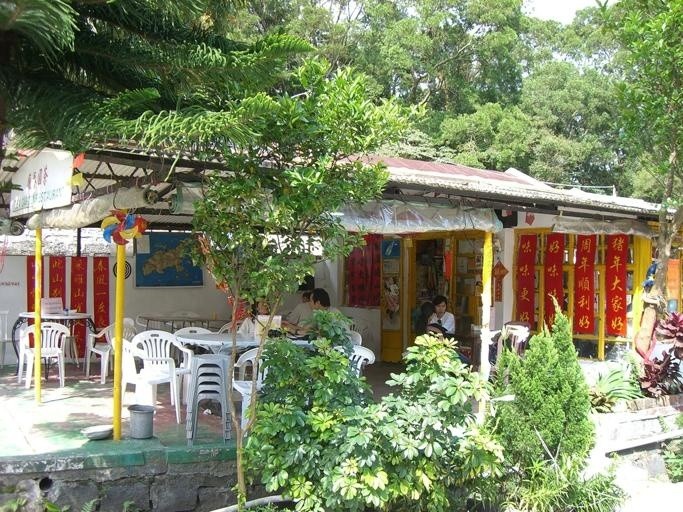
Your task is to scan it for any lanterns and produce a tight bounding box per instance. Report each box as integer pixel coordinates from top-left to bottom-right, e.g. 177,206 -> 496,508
135,216 -> 148,232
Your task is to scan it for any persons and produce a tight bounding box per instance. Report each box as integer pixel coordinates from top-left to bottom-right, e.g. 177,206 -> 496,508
414,295 -> 456,340
237,275 -> 352,348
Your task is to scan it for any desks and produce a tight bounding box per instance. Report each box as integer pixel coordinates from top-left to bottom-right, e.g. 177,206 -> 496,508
12,311 -> 96,382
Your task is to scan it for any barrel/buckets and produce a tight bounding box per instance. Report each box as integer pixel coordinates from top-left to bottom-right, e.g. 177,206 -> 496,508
129,404 -> 155,440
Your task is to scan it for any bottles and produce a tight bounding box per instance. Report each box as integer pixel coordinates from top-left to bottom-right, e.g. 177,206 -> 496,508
64,308 -> 69,316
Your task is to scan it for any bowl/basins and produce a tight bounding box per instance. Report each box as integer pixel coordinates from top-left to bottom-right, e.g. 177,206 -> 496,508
80,425 -> 113,440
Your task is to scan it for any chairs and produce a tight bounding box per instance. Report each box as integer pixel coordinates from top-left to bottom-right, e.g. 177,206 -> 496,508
18,322 -> 70,390
86,311 -> 376,444
